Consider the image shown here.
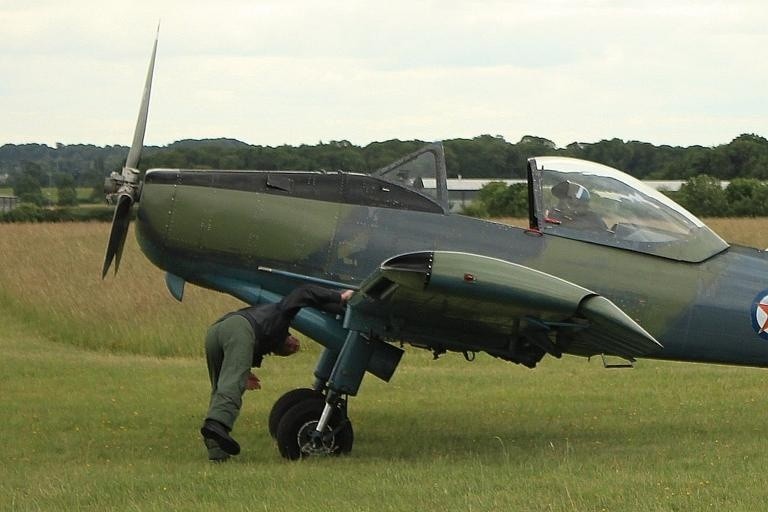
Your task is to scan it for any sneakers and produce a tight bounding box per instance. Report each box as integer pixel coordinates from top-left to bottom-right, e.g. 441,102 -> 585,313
201,419 -> 240,462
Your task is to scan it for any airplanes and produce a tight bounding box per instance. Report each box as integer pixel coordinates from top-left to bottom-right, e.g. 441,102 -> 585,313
96,32 -> 768,458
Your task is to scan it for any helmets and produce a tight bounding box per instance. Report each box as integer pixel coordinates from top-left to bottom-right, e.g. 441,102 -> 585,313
548,180 -> 590,220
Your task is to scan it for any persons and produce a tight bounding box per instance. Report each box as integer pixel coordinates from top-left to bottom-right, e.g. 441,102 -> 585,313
201,284 -> 355,462
551,181 -> 610,237
